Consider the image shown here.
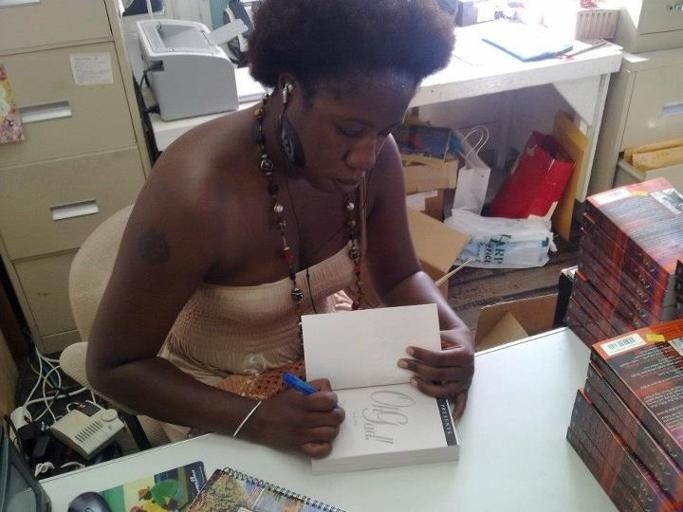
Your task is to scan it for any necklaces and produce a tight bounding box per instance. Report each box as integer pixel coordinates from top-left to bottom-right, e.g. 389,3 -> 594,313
254,96 -> 365,355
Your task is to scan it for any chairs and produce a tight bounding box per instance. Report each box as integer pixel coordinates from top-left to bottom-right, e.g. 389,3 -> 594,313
56,201 -> 149,462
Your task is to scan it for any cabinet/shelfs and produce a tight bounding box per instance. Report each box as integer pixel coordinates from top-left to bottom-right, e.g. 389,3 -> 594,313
599,2 -> 683,53
0,0 -> 153,354
588,47 -> 682,195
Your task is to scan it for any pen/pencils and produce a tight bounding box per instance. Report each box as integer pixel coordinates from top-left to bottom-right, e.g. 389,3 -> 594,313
282,373 -> 319,394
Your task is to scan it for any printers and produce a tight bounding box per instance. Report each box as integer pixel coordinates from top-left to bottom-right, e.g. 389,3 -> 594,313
136,16 -> 249,122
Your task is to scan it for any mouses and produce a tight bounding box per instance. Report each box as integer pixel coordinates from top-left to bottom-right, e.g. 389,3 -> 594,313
67,491 -> 111,512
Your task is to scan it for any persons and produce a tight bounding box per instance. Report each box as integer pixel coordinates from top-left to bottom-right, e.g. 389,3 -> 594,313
81,1 -> 479,461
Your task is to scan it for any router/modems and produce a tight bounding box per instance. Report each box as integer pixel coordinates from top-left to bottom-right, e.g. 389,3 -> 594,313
51,401 -> 126,458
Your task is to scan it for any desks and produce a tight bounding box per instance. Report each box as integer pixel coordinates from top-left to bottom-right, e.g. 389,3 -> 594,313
124,2 -> 626,259
40,325 -> 624,512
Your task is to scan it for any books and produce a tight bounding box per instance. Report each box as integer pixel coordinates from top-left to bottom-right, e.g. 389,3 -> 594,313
391,122 -> 454,168
565,319 -> 682,511
561,176 -> 683,350
296,302 -> 461,477
185,465 -> 349,511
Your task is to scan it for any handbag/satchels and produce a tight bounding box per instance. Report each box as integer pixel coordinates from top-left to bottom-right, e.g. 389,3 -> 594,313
487,130 -> 576,218
442,125 -> 492,222
